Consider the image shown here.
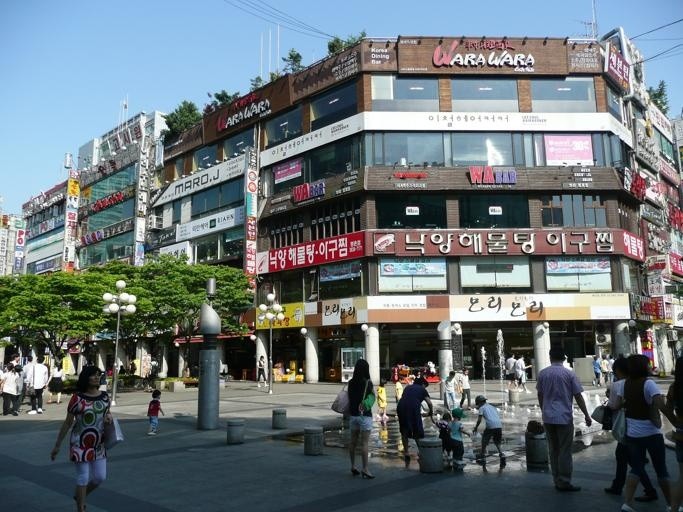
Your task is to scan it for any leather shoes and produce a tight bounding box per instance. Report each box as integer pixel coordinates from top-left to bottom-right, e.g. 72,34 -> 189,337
555,485 -> 581,491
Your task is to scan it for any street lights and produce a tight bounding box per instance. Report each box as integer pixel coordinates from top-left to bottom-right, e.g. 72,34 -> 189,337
16,324 -> 26,366
101,279 -> 137,406
206,277 -> 217,309
258,292 -> 287,395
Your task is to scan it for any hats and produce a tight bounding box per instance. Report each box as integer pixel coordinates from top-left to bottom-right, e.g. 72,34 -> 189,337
476,395 -> 487,403
452,408 -> 465,418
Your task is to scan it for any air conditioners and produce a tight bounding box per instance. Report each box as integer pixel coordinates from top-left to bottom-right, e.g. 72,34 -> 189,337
595,334 -> 611,345
595,322 -> 611,334
668,330 -> 678,341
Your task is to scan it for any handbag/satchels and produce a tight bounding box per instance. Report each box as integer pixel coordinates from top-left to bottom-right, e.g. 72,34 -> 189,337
611,408 -> 626,443
527,421 -> 544,433
591,406 -> 604,424
104,418 -> 125,448
361,393 -> 374,410
26,384 -> 35,395
331,390 -> 350,413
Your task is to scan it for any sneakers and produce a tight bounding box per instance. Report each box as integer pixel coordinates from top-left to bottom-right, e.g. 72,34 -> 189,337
442,453 -> 506,464
605,487 -> 683,512
375,414 -> 380,418
3,408 -> 42,416
383,414 -> 388,418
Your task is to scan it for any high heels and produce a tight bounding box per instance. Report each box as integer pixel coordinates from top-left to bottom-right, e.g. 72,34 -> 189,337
351,469 -> 360,476
362,472 -> 375,479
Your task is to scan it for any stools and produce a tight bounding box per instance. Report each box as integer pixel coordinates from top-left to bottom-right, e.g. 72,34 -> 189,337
227,419 -> 246,443
272,409 -> 287,429
526,431 -> 549,468
419,436 -> 444,473
305,427 -> 324,455
509,388 -> 520,403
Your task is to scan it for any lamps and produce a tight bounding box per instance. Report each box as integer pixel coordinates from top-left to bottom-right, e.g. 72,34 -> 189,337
300,328 -> 308,339
454,323 -> 460,333
541,322 -> 549,332
250,335 -> 257,344
361,324 -> 370,336
388,157 -> 405,179
163,148 -> 245,185
83,139 -> 145,181
623,320 -> 636,334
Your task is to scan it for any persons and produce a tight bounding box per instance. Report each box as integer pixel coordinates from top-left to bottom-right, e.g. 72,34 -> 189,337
349,352 -> 533,478
536,348 -> 592,490
605,356 -> 656,503
120,366 -> 126,373
592,353 -> 615,386
258,355 -> 268,387
147,389 -> 165,435
621,354 -> 673,512
131,362 -> 135,374
149,357 -> 158,377
50,366 -> 111,511
0,354 -> 48,416
665,355 -> 683,512
47,361 -> 66,404
